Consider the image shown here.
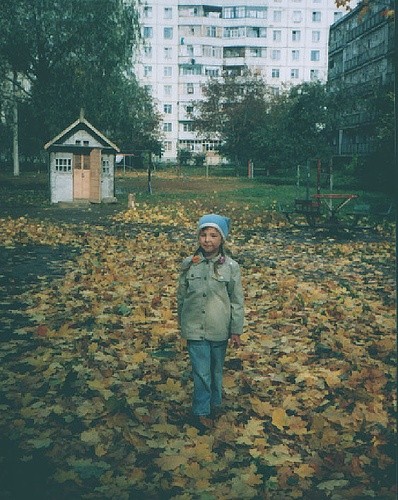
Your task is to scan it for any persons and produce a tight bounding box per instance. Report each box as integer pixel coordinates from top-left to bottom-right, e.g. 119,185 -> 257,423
177,213 -> 244,430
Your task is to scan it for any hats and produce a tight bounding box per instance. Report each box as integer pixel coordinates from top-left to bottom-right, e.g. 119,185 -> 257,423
197,214 -> 231,241
